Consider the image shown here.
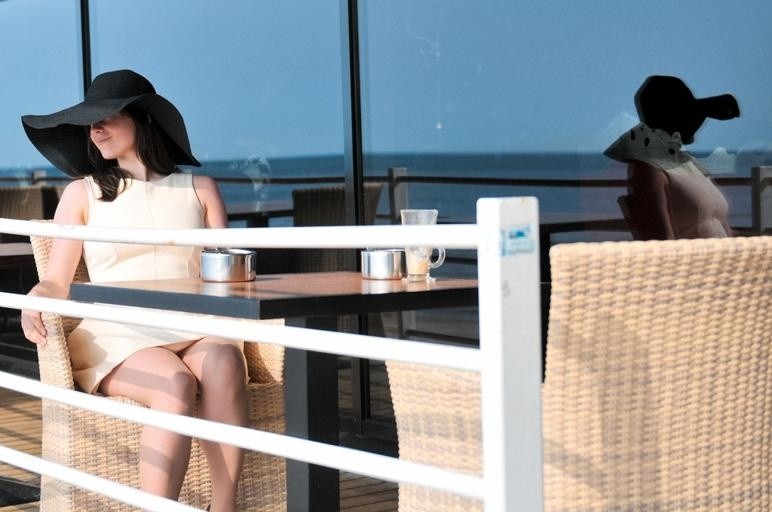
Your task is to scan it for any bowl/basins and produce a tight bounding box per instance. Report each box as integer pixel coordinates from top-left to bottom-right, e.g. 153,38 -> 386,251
361,251 -> 403,281
202,248 -> 257,282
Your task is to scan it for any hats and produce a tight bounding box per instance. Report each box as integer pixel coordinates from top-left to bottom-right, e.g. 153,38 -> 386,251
604,75 -> 740,164
21,69 -> 202,177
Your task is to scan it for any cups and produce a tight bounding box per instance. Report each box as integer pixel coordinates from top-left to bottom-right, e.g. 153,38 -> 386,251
402,208 -> 445,282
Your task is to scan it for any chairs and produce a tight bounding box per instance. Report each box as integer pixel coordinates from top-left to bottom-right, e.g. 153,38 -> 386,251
379,234 -> 759,511
3,189 -> 71,316
22,217 -> 292,511
247,183 -> 382,274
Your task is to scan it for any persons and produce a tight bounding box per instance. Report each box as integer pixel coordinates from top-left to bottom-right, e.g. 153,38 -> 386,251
17,68 -> 252,512
601,74 -> 742,241
